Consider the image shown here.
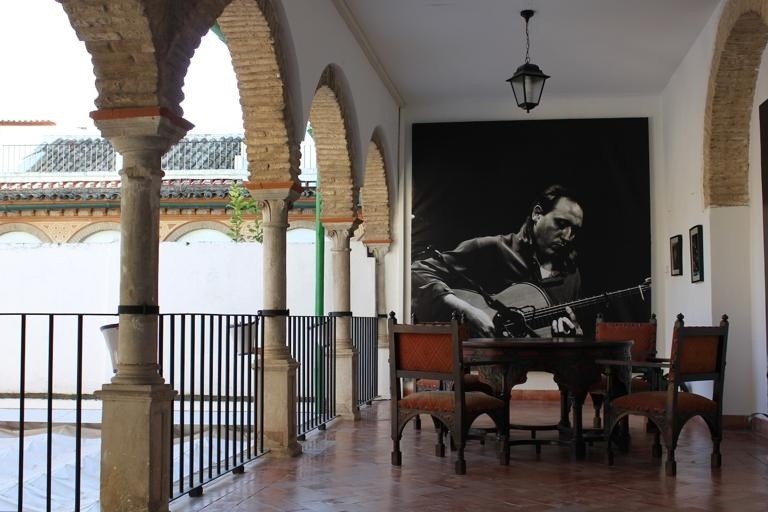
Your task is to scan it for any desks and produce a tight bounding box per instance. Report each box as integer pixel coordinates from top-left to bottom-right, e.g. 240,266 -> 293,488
430,336 -> 635,466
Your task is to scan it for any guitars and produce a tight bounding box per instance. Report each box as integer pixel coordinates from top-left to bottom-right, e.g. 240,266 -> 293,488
430,277 -> 651,338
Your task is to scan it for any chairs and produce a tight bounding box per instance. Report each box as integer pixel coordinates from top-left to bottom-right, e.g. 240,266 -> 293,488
595,313 -> 729,476
593,313 -> 657,432
388,311 -> 511,475
411,312 -> 479,429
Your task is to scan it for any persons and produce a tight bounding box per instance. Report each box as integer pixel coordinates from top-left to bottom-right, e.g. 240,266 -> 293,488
411,182 -> 592,340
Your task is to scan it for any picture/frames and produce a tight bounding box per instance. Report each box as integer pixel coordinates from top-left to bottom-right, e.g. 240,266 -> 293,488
669,235 -> 682,276
689,225 -> 704,283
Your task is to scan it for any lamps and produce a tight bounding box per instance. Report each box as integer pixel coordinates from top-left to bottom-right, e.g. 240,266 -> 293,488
506,10 -> 550,114
